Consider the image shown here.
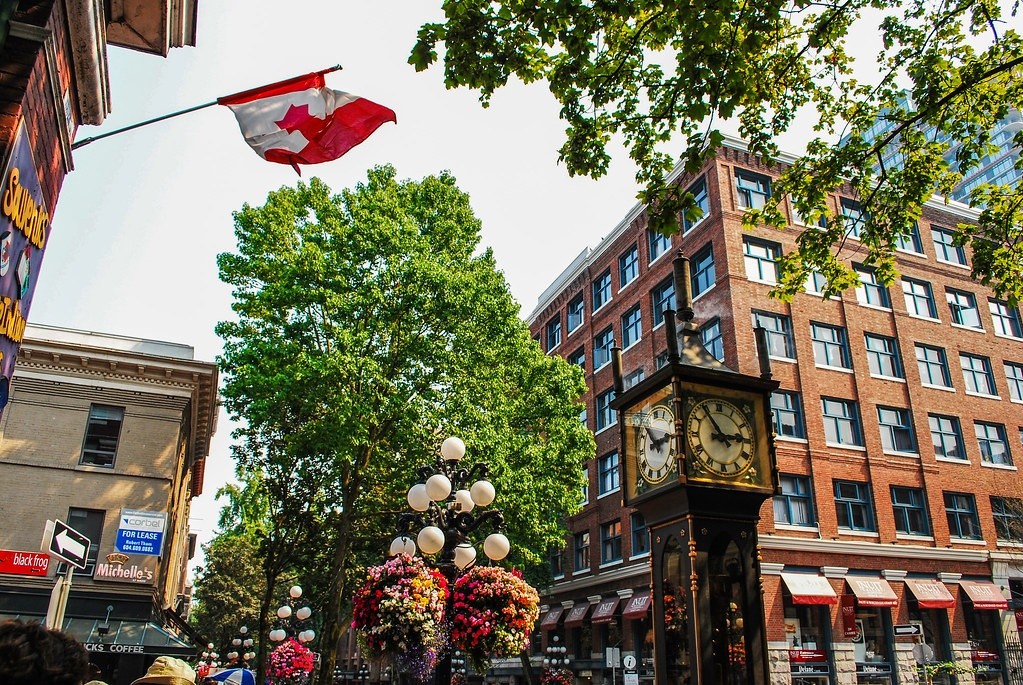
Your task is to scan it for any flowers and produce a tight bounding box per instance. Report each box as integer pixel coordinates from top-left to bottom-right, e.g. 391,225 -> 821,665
450,566 -> 542,664
350,553 -> 453,684
663,595 -> 687,631
728,644 -> 747,667
541,667 -> 573,685
264,636 -> 316,685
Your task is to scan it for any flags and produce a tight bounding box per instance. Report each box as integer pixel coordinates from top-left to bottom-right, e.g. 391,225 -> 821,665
216,71 -> 397,176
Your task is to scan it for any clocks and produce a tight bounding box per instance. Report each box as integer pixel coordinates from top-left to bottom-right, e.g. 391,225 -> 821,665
623,383 -> 681,502
681,379 -> 775,494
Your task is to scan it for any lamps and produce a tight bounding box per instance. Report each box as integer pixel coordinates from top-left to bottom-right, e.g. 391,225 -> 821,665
98,605 -> 114,634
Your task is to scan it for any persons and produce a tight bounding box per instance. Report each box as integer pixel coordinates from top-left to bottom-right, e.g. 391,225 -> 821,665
0,619 -> 197,685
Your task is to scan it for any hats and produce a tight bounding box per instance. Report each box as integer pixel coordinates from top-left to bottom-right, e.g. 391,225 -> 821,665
130,656 -> 196,685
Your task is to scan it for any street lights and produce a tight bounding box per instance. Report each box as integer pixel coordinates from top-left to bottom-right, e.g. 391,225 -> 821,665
358,665 -> 369,685
389,437 -> 510,685
543,636 -> 569,670
227,625 -> 256,668
333,665 -> 342,685
451,650 -> 465,674
198,642 -> 221,668
269,585 -> 315,643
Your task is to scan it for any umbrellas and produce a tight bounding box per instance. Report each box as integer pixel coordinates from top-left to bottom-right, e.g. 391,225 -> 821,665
214,668 -> 269,685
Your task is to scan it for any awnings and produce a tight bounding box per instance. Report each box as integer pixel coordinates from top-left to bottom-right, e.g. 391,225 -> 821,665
846,576 -> 897,607
564,602 -> 590,628
540,607 -> 564,630
781,573 -> 837,604
961,581 -> 1007,609
591,597 -> 620,623
623,590 -> 653,619
906,579 -> 956,608
